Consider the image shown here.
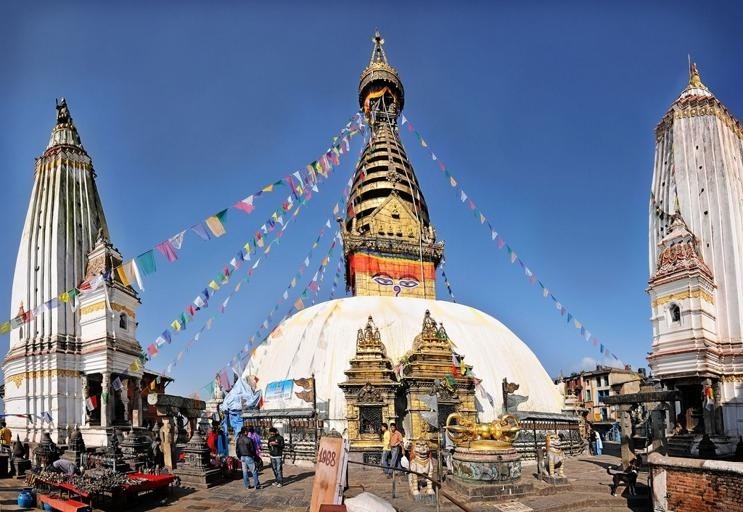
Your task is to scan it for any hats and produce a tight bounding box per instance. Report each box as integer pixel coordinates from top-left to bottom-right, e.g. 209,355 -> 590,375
79,466 -> 85,477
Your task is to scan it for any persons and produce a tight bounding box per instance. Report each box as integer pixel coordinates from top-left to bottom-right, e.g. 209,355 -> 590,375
381,423 -> 391,474
387,423 -> 406,479
0,422 -> 12,460
151,438 -> 163,470
207,425 -> 284,489
46,459 -> 82,476
590,430 -> 603,455
699,434 -> 717,460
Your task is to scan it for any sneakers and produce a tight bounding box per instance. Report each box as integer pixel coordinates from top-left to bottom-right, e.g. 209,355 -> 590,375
272,481 -> 282,488
246,485 -> 261,490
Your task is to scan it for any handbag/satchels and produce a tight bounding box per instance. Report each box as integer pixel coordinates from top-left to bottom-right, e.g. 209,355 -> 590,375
400,456 -> 410,468
